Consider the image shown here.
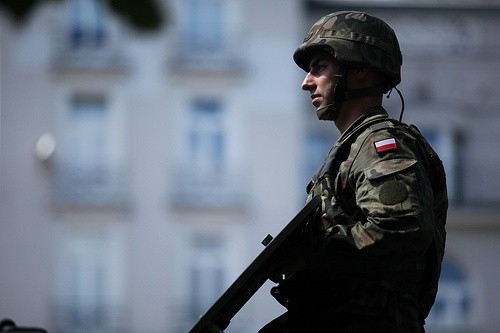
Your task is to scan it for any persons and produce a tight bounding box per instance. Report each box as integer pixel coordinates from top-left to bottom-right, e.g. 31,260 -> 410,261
251,8 -> 450,332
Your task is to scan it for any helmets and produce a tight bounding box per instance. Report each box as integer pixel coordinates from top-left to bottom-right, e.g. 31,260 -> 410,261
293,10 -> 403,95
